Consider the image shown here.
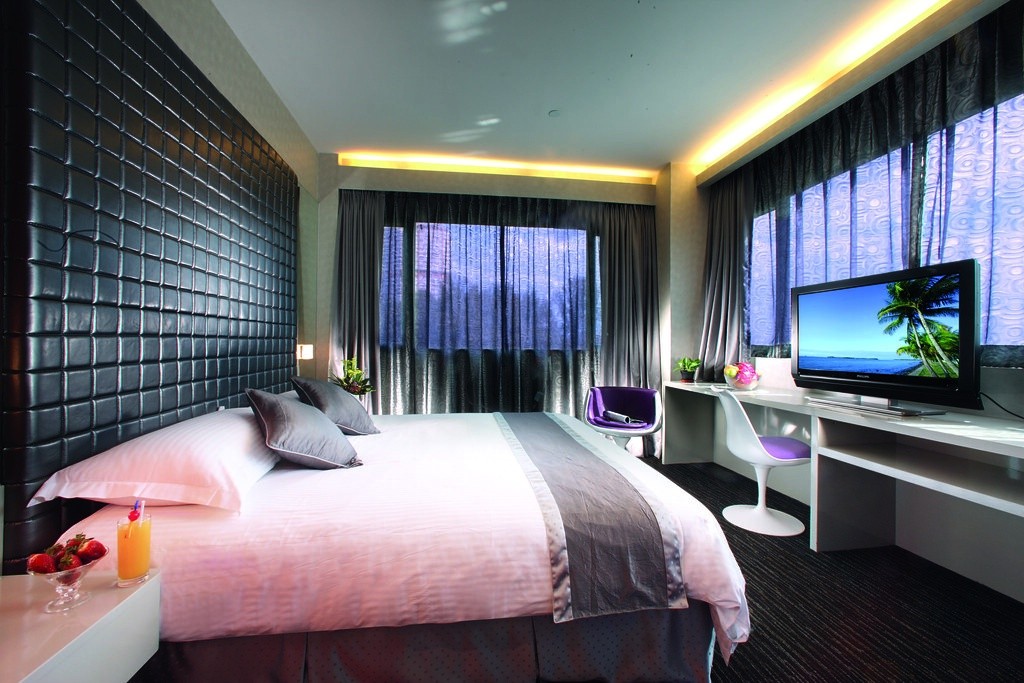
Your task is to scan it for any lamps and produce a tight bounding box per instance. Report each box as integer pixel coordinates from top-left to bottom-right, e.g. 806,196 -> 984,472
297,344 -> 313,360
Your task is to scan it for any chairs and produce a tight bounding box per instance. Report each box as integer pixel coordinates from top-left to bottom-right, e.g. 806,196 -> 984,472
583,386 -> 663,454
710,385 -> 811,537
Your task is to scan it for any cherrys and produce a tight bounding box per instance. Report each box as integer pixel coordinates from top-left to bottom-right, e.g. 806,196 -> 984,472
128,509 -> 141,522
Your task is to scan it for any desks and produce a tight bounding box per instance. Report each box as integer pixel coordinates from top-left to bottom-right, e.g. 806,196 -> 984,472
670,380 -> 1024,604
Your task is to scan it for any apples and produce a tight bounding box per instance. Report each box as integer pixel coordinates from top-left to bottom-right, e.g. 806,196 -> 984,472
723,365 -> 738,378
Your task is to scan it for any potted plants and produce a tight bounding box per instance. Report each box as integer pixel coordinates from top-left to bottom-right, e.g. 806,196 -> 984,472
328,358 -> 376,395
674,357 -> 702,380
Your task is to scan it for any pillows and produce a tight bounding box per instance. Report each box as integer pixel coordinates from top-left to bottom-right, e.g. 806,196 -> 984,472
27,390 -> 301,513
292,376 -> 380,436
245,388 -> 363,470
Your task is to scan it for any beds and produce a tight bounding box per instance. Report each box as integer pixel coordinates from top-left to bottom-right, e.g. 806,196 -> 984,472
1,0 -> 754,683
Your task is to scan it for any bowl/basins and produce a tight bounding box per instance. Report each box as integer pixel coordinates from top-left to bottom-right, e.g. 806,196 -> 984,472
723,376 -> 762,390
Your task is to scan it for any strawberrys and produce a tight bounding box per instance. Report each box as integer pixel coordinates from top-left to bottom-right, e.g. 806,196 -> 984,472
25,533 -> 107,585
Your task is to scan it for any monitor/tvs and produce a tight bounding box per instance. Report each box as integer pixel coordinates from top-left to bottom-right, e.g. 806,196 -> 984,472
790,258 -> 985,417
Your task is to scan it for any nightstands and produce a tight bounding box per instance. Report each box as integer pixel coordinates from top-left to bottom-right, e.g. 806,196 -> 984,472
0,569 -> 162,682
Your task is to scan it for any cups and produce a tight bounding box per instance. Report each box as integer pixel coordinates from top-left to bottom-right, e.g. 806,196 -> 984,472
116,514 -> 152,588
